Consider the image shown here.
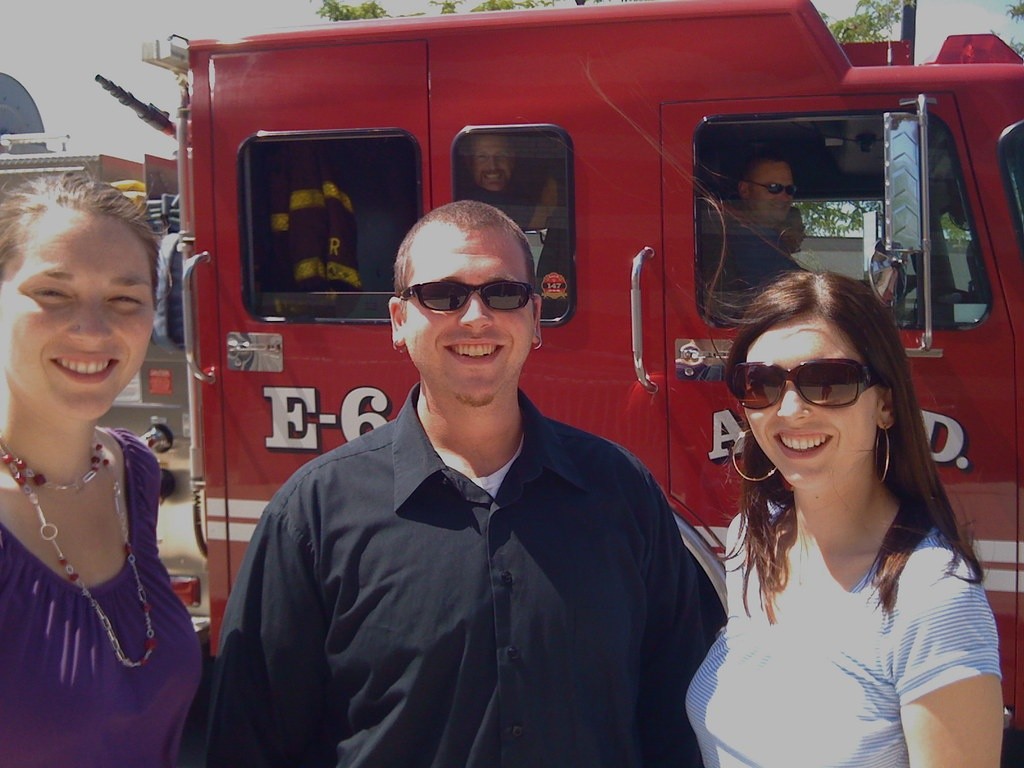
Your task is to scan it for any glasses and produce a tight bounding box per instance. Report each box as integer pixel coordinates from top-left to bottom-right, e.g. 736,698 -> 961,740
729,359 -> 882,410
400,280 -> 533,313
746,180 -> 797,196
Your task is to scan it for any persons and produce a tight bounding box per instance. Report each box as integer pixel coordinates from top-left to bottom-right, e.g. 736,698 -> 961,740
709,155 -> 913,330
459,133 -> 560,229
0,173 -> 205,768
685,274 -> 1004,768
172,200 -> 727,768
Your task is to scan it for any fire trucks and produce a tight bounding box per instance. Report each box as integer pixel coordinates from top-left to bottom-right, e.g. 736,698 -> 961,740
0,0 -> 1024,734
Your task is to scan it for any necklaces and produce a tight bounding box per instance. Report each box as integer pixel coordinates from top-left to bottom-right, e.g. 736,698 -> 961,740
0,438 -> 158,668
